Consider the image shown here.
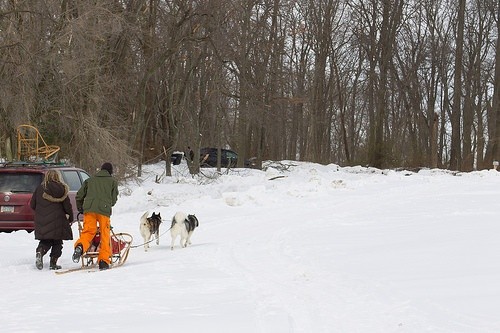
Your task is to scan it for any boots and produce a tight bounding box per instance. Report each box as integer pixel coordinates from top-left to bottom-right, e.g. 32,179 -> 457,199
36,246 -> 47,270
50,255 -> 61,270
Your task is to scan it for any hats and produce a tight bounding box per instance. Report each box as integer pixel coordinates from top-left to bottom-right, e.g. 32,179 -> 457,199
101,162 -> 112,175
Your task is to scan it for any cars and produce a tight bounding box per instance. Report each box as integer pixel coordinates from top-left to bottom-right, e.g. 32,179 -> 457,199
199,147 -> 255,168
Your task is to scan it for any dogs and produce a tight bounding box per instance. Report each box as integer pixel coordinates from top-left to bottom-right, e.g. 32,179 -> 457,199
140,211 -> 161,252
170,212 -> 199,251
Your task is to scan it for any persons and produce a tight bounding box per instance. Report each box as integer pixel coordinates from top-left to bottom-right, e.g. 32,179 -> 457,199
30,170 -> 74,270
72,163 -> 119,270
188,148 -> 194,163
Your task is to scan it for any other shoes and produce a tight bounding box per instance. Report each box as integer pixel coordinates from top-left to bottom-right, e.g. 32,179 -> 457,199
99,260 -> 109,270
72,243 -> 83,263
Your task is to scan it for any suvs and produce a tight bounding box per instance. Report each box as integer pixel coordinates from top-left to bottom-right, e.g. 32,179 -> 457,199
0,161 -> 92,233
